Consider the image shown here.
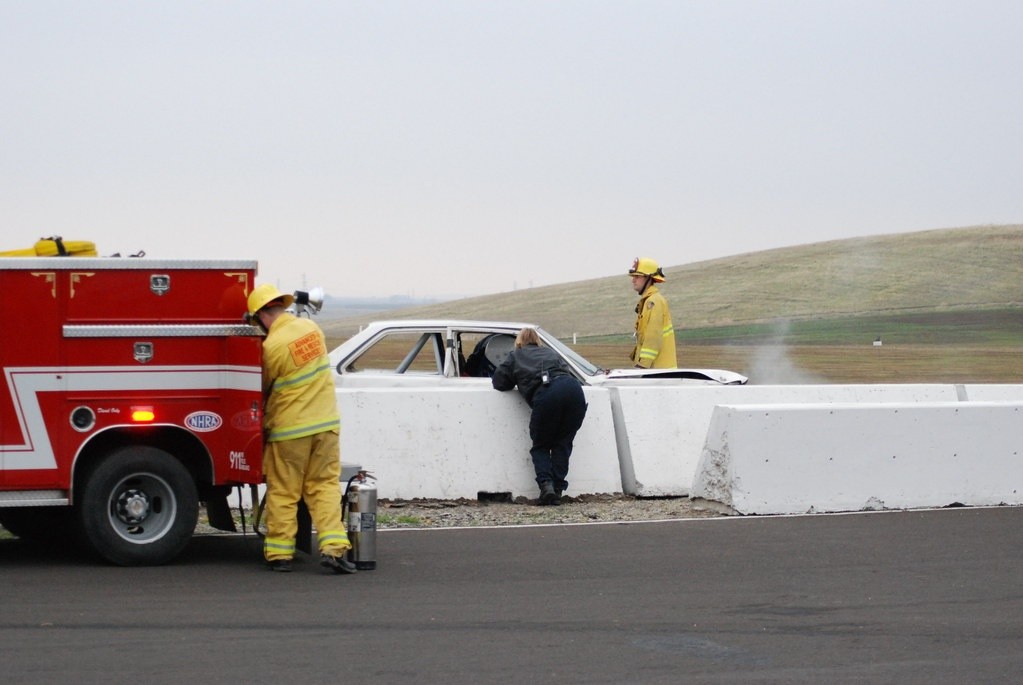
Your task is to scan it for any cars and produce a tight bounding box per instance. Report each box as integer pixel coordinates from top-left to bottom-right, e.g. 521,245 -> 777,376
323,321 -> 748,387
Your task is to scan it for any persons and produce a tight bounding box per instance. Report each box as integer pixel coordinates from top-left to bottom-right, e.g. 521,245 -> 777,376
492,327 -> 588,505
627,257 -> 678,368
246,283 -> 357,574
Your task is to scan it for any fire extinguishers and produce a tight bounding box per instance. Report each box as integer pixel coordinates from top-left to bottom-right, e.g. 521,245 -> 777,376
341,470 -> 378,571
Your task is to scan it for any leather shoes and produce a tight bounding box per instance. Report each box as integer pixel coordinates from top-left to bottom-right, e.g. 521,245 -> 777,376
539,481 -> 562,506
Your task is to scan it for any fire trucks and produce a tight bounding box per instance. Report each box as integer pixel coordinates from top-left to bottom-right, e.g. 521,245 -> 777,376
0,235 -> 264,567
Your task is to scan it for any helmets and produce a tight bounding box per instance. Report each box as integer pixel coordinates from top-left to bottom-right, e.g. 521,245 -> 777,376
247,282 -> 295,318
628,256 -> 666,283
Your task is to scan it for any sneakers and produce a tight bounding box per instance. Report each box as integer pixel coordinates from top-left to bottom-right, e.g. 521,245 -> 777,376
264,557 -> 293,571
319,552 -> 357,574
